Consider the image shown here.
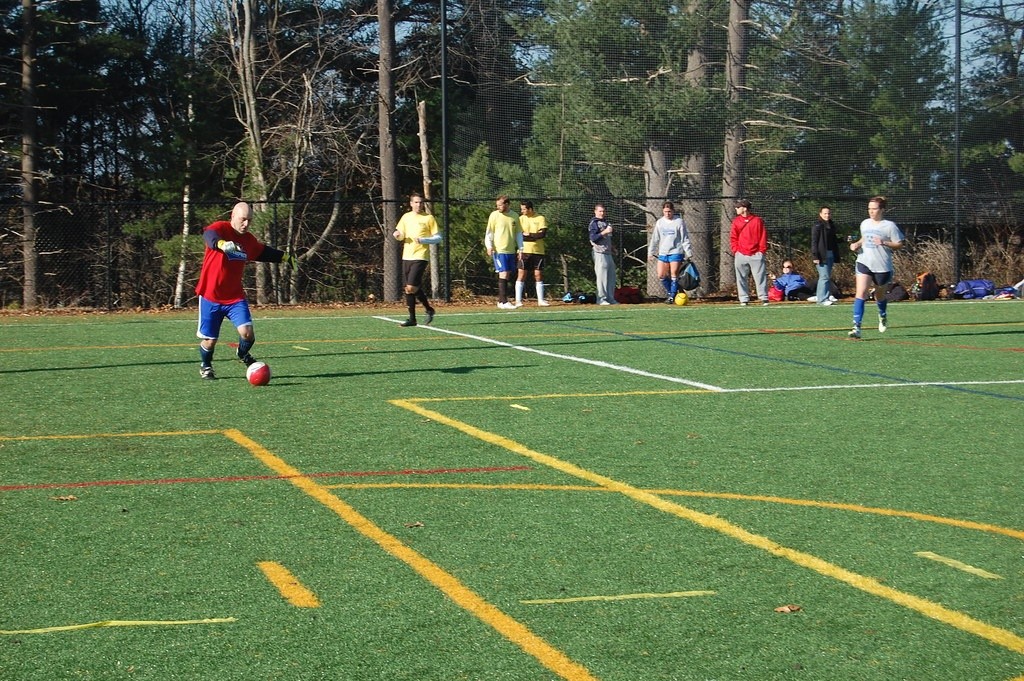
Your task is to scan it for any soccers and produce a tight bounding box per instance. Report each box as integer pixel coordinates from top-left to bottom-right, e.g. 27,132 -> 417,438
246,361 -> 272,386
675,292 -> 689,306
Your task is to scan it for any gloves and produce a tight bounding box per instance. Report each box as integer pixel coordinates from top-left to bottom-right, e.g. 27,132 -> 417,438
283,252 -> 303,270
220,241 -> 239,253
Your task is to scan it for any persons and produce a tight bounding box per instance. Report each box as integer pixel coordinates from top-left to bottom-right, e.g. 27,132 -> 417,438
514,198 -> 549,307
485,194 -> 524,309
393,192 -> 444,327
588,203 -> 622,305
849,195 -> 905,339
648,201 -> 693,304
195,203 -> 297,380
770,260 -> 838,302
810,207 -> 852,305
730,200 -> 770,307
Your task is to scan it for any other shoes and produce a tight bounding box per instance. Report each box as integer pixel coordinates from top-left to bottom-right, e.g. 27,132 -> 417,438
600,300 -> 611,306
879,323 -> 888,333
424,307 -> 435,325
515,302 -> 524,307
400,318 -> 418,326
807,296 -> 818,302
537,300 -> 550,307
498,301 -> 518,309
236,348 -> 257,368
199,361 -> 215,380
664,296 -> 674,304
740,302 -> 748,305
761,300 -> 770,305
817,299 -> 833,306
848,326 -> 862,338
829,296 -> 838,301
612,301 -> 621,304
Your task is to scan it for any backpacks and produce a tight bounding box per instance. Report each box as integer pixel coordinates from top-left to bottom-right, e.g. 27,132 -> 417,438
912,272 -> 940,301
675,259 -> 701,291
951,279 -> 995,300
574,282 -> 646,304
871,281 -> 909,302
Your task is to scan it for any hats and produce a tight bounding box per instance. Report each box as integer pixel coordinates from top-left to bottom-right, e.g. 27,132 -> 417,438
735,199 -> 752,209
